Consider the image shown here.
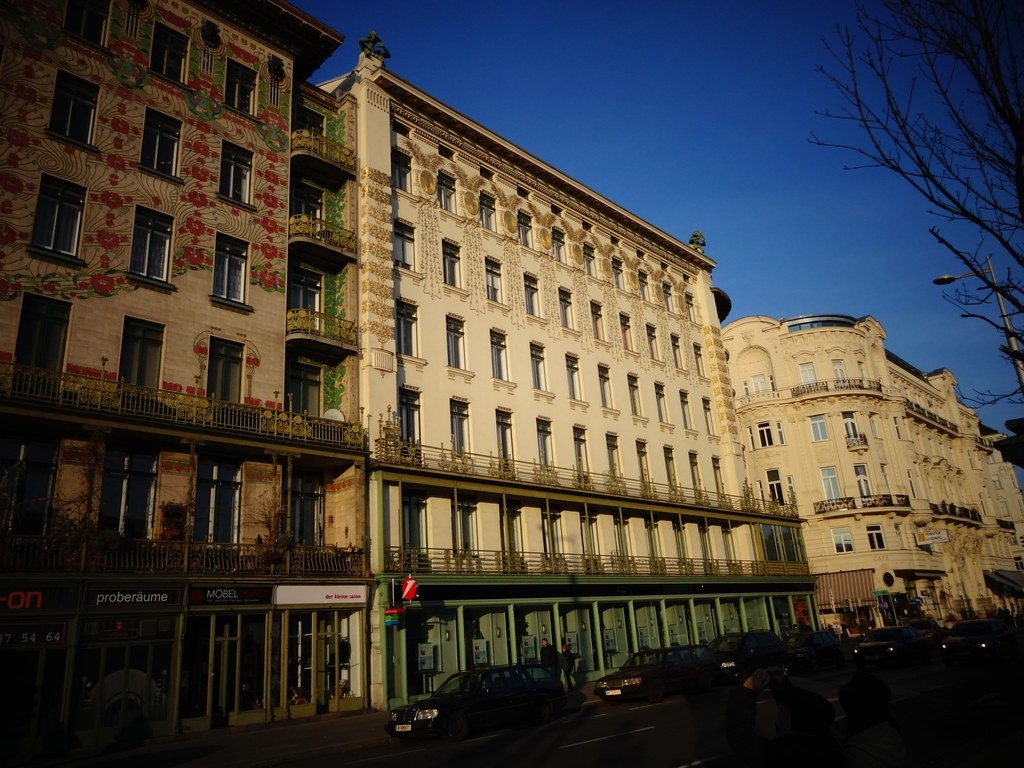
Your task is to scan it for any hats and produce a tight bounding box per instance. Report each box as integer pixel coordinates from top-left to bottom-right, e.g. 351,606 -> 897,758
771,687 -> 836,735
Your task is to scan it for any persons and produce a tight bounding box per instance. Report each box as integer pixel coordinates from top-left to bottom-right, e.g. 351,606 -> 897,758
725,656 -> 912,768
562,644 -> 574,689
840,622 -> 849,639
789,621 -> 813,637
997,606 -> 1010,619
540,638 -> 555,673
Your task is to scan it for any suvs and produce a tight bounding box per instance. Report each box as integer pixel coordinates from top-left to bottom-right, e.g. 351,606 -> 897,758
384,663 -> 568,744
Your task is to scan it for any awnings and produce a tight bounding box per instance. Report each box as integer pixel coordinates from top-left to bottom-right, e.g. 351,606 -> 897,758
983,570 -> 1024,596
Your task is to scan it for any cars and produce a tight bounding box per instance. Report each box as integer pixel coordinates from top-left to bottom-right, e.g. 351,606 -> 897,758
780,631 -> 846,672
906,617 -> 943,649
693,629 -> 786,690
592,642 -> 708,703
941,618 -> 1017,671
852,626 -> 927,672
